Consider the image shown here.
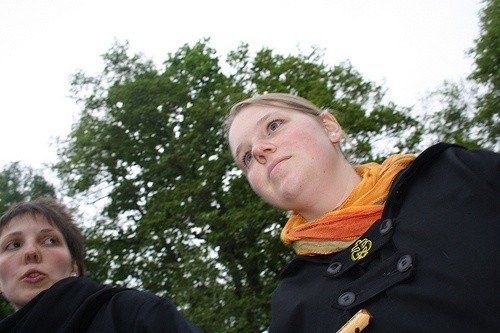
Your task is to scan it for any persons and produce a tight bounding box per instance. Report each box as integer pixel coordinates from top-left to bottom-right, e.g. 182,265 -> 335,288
0,199 -> 197,333
229,93 -> 499,333
